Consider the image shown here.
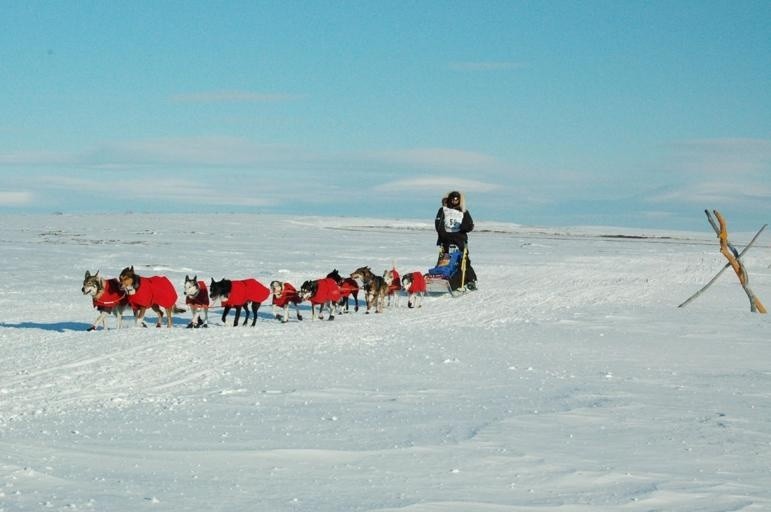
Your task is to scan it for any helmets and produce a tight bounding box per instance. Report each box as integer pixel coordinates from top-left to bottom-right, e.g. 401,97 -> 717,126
447,192 -> 460,208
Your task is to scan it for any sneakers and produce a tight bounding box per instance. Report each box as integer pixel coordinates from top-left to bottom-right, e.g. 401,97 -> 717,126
468,281 -> 476,289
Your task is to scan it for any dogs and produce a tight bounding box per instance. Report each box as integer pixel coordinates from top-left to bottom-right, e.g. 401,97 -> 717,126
270,280 -> 303,323
349,266 -> 390,314
326,269 -> 360,314
209,277 -> 270,327
402,272 -> 426,308
118,265 -> 187,329
183,275 -> 210,328
81,269 -> 131,332
382,255 -> 402,308
298,278 -> 343,322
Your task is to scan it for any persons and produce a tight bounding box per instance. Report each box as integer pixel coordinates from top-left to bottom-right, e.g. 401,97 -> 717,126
435,190 -> 479,292
438,193 -> 468,242
